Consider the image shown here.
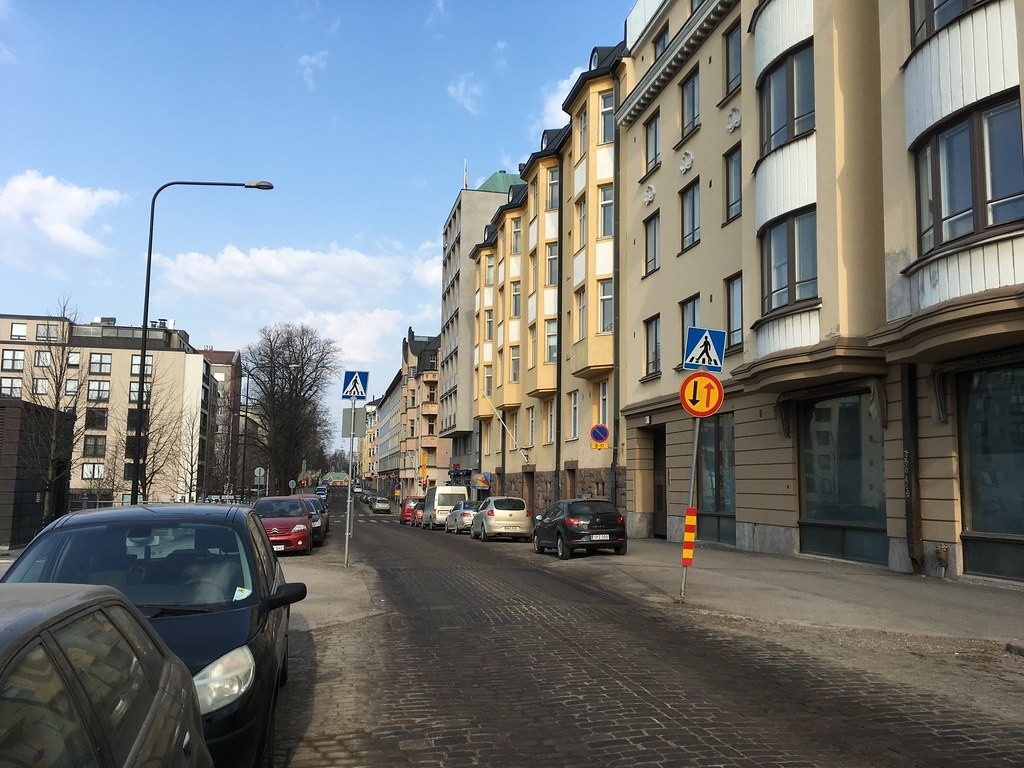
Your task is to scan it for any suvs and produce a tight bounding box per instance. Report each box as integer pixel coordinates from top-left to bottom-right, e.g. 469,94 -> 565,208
236,485 -> 258,495
372,497 -> 391,514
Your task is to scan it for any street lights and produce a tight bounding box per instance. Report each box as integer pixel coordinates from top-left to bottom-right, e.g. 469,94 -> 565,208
240,363 -> 302,504
130,180 -> 276,505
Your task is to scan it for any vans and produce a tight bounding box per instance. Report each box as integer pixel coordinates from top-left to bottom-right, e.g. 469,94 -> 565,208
399,495 -> 425,525
421,485 -> 468,530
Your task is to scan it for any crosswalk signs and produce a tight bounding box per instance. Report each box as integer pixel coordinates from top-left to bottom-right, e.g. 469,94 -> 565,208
682,326 -> 727,374
342,370 -> 370,400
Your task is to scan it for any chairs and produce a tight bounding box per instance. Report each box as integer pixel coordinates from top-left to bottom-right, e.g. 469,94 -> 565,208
261,504 -> 274,513
288,504 -> 299,514
166,527 -> 243,609
85,530 -> 137,585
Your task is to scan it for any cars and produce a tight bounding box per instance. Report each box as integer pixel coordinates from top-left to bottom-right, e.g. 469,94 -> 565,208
351,483 -> 378,510
410,502 -> 425,527
470,496 -> 533,541
445,501 -> 483,534
1,503 -> 308,768
252,495 -> 313,555
304,498 -> 325,547
531,498 -> 628,561
0,581 -> 216,768
289,479 -> 330,532
